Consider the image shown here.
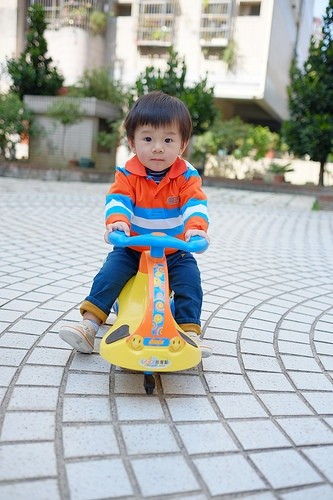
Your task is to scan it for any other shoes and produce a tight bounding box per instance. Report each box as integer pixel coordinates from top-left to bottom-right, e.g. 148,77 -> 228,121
185,331 -> 213,358
59,319 -> 100,354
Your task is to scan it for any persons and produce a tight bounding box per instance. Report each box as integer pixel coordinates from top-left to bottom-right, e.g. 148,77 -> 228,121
58,91 -> 211,357
